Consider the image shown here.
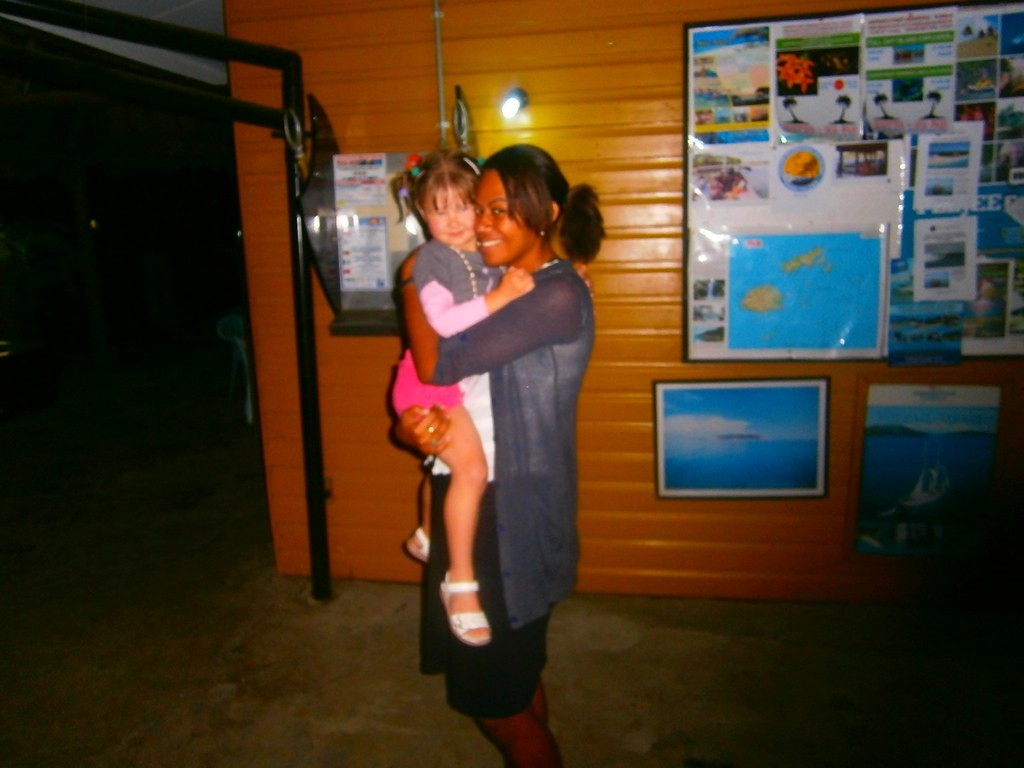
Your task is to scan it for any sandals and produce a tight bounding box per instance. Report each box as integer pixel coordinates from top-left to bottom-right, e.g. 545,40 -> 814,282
407,527 -> 429,562
438,571 -> 492,646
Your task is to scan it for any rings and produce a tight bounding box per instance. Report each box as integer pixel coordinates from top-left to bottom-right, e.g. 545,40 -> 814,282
429,427 -> 435,433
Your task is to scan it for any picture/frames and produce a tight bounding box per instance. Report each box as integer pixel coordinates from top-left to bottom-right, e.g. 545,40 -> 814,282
652,374 -> 828,499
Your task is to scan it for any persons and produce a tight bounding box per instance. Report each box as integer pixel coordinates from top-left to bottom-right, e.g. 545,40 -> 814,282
388,145 -> 607,767
391,150 -> 535,646
